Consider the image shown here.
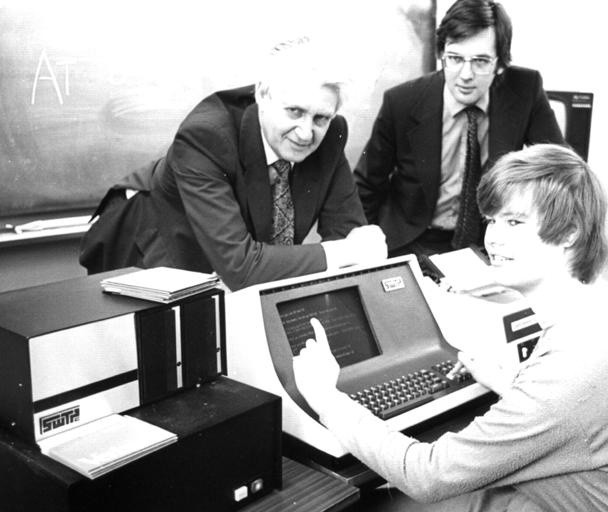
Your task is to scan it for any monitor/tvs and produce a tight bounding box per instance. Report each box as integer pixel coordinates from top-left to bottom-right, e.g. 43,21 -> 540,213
276,285 -> 380,367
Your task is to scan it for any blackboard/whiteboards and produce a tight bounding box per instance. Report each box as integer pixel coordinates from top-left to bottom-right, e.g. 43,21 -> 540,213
0,0 -> 438,245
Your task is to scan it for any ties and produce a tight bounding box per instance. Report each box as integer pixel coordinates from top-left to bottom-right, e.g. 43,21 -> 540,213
271,158 -> 295,247
453,107 -> 482,253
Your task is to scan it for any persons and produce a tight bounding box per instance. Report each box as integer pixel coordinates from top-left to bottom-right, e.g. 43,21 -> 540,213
79,79 -> 391,292
351,0 -> 576,282
290,144 -> 608,512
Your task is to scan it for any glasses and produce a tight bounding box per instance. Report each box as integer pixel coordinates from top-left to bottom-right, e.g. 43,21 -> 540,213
440,53 -> 501,74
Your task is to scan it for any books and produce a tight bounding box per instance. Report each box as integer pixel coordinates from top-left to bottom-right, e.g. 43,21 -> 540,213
98,265 -> 220,304
41,412 -> 179,481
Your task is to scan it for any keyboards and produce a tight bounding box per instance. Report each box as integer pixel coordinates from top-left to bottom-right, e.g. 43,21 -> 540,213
346,359 -> 476,420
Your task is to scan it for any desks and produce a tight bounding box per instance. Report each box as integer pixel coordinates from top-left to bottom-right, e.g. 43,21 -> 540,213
240,453 -> 361,511
